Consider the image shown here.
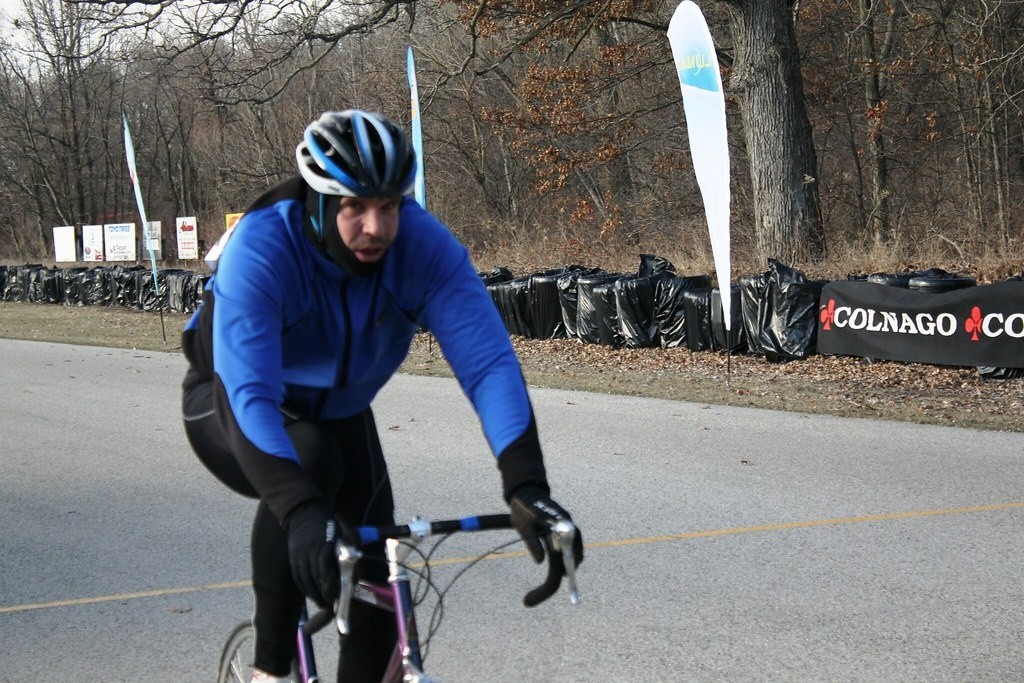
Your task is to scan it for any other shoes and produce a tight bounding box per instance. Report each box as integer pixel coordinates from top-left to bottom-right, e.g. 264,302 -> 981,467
246,665 -> 294,683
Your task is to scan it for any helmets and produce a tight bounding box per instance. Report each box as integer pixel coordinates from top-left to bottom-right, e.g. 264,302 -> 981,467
294,106 -> 419,200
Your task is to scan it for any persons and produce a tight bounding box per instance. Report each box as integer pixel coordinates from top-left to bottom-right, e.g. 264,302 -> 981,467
182,108 -> 584,682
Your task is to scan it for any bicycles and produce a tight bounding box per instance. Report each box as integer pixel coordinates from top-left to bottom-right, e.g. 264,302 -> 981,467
216,505 -> 586,683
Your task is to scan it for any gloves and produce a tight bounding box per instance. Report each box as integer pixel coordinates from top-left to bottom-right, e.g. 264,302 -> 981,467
510,493 -> 584,575
287,507 -> 359,602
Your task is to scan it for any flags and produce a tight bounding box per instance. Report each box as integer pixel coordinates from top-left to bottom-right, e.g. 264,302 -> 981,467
668,0 -> 729,331
408,47 -> 425,210
